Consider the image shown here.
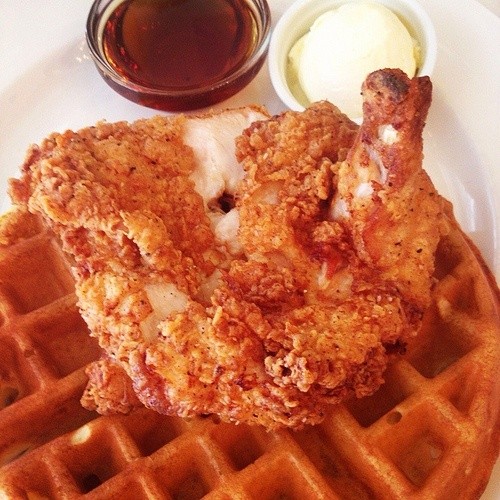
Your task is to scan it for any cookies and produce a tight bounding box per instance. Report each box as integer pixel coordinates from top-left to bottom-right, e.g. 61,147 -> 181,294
0,200 -> 500,500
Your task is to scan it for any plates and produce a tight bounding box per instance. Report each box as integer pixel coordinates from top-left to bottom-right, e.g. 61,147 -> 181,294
2,1 -> 500,296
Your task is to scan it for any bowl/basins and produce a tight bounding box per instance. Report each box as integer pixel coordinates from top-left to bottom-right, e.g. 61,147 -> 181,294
85,1 -> 272,113
266,1 -> 436,125
